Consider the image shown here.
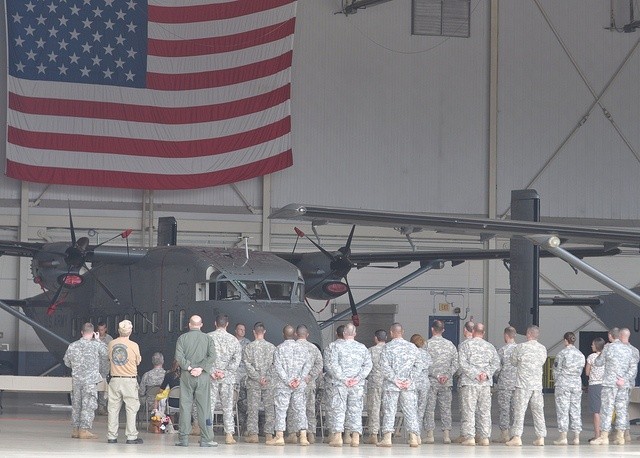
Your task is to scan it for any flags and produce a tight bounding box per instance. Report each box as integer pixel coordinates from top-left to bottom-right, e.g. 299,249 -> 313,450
3,1 -> 298,189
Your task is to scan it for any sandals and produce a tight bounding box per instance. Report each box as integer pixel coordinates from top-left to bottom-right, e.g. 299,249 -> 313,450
588,437 -> 597,442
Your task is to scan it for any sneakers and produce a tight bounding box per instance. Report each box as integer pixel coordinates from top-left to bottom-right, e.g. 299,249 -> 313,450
492,431 -> 510,442
108,439 -> 117,443
553,432 -> 567,445
127,439 -> 143,444
175,441 -> 188,446
199,441 -> 218,447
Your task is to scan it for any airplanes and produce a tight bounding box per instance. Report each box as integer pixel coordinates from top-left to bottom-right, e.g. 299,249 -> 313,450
266,189 -> 640,335
0,196 -> 623,412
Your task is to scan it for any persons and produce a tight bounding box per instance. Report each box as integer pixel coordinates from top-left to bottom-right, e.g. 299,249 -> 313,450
505,325 -> 548,446
453,322 -> 480,443
190,399 -> 200,435
175,314 -> 217,447
159,359 -> 181,408
322,325 -> 352,443
590,327 -> 636,445
106,320 -> 144,443
206,314 -> 237,443
608,327 -> 639,441
364,330 -> 387,444
139,352 -> 175,424
91,322 -> 114,416
329,323 -> 373,447
492,325 -> 519,443
422,319 -> 459,444
410,334 -> 433,444
376,322 -> 422,447
552,332 -> 585,445
285,324 -> 324,443
63,322 -> 110,438
459,323 -> 500,445
243,322 -> 279,442
266,324 -> 311,445
585,337 -> 605,441
234,323 -> 252,429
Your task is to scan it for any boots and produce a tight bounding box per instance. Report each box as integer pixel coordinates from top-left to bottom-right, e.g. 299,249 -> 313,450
613,431 -> 625,445
285,433 -> 297,444
343,430 -> 352,444
570,432 -> 579,445
352,432 -> 359,447
452,436 -> 467,443
191,424 -> 200,435
244,434 -> 259,443
225,433 -> 236,444
422,430 -> 434,444
72,430 -> 78,438
462,436 -> 475,446
478,437 -> 489,446
265,434 -> 273,441
79,430 -> 98,439
443,430 -> 451,444
590,432 -> 609,445
300,429 -> 310,446
323,433 -> 333,442
363,434 -> 377,444
625,430 -> 632,441
329,432 -> 343,446
506,435 -> 522,446
376,432 -> 392,447
533,436 -> 544,446
308,433 -> 315,443
409,433 -> 418,447
265,431 -> 285,446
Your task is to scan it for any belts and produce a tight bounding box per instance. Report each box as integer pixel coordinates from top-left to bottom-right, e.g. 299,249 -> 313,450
111,375 -> 138,378
